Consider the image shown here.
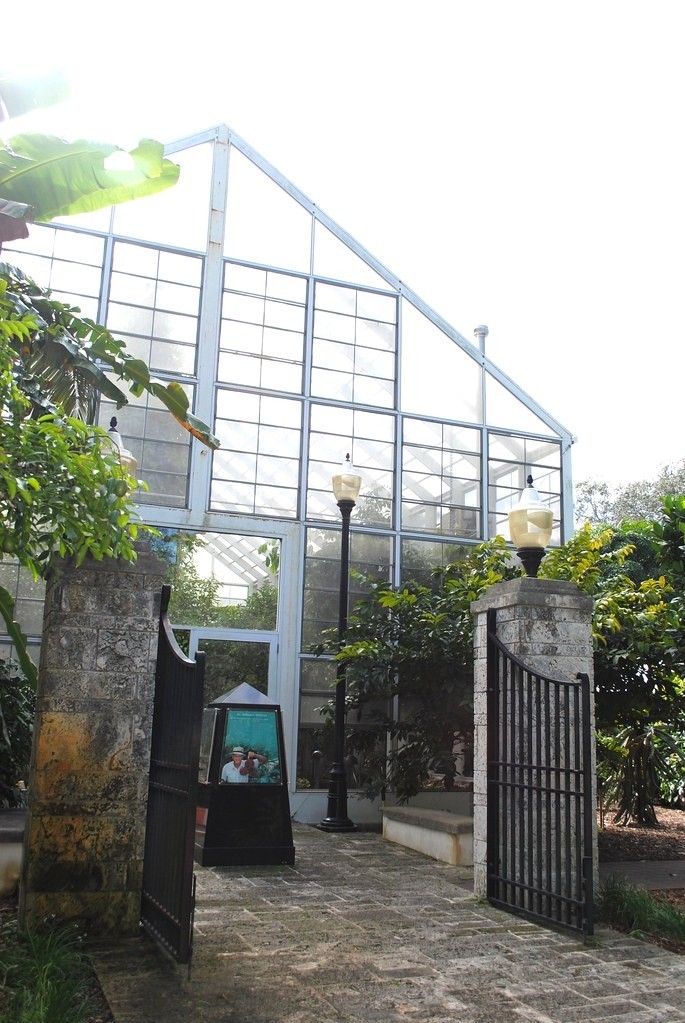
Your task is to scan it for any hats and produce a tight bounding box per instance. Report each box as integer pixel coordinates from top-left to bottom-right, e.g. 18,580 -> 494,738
228,747 -> 246,759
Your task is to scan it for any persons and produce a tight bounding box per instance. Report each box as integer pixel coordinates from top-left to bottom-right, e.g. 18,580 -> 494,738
220,747 -> 266,784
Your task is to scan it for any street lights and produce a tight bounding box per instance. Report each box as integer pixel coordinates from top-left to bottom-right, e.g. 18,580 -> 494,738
316,452 -> 365,833
